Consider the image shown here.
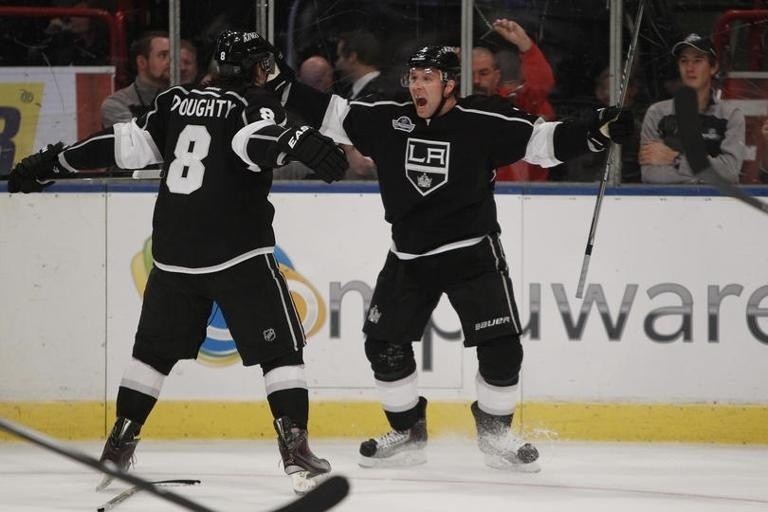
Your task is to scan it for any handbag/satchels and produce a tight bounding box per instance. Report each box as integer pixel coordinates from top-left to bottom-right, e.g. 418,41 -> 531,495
656,113 -> 729,160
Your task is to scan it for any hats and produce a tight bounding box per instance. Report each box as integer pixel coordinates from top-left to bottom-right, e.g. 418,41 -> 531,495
670,30 -> 716,56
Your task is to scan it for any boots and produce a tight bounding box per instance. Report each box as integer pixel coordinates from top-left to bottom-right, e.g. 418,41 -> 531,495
272,417 -> 331,496
357,395 -> 429,470
95,415 -> 142,491
470,400 -> 542,475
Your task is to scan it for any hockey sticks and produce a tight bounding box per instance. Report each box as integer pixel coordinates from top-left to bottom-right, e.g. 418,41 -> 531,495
0,420 -> 349,512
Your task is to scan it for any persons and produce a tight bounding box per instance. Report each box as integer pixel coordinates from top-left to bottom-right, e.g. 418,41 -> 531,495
637,29 -> 744,184
178,37 -> 207,88
99,34 -> 170,179
272,57 -> 375,181
8,33 -> 349,495
267,45 -> 633,472
336,30 -> 412,99
550,68 -> 642,182
36,4 -> 106,64
456,20 -> 556,180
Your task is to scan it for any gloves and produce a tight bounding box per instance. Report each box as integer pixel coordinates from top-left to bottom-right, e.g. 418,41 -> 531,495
279,123 -> 350,185
578,103 -> 632,156
6,140 -> 73,195
265,39 -> 291,103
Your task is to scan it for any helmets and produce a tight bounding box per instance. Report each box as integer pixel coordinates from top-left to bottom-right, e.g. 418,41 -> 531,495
400,44 -> 463,89
215,28 -> 276,77
